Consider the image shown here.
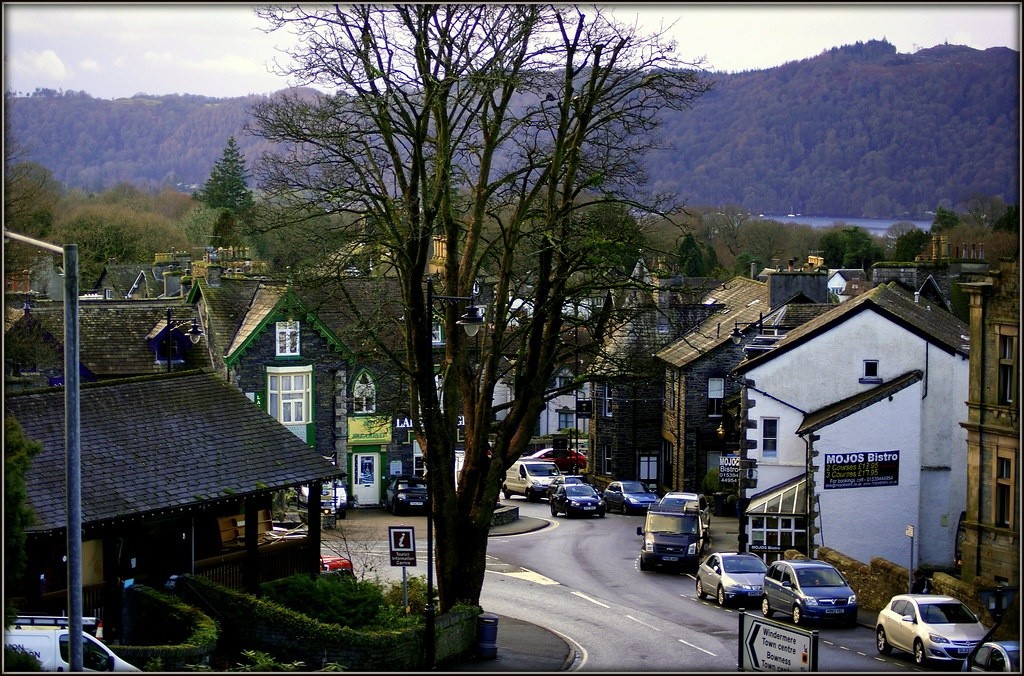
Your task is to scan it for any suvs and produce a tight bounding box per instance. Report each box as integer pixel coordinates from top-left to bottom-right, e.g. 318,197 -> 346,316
658,491 -> 710,531
320,554 -> 352,577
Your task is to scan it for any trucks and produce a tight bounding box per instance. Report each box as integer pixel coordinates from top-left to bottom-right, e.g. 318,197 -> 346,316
637,502 -> 706,573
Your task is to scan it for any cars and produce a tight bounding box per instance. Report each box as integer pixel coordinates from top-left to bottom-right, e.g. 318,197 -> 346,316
546,475 -> 603,500
387,476 -> 431,514
876,594 -> 987,665
960,641 -> 1020,672
603,479 -> 662,514
533,447 -> 587,472
696,552 -> 771,607
551,483 -> 606,518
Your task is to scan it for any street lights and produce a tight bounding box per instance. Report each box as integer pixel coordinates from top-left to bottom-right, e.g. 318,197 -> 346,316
167,306 -> 203,373
422,277 -> 486,672
732,310 -> 763,345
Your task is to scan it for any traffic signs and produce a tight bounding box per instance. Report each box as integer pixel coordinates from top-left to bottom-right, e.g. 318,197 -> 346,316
741,616 -> 812,673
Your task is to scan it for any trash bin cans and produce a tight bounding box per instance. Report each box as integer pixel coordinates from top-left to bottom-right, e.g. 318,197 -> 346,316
712,491 -> 731,518
474,614 -> 499,660
321,506 -> 337,529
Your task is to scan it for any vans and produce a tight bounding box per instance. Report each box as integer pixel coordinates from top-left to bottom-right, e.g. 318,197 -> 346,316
3,614 -> 142,673
296,478 -> 347,519
501,457 -> 562,501
761,558 -> 858,626
